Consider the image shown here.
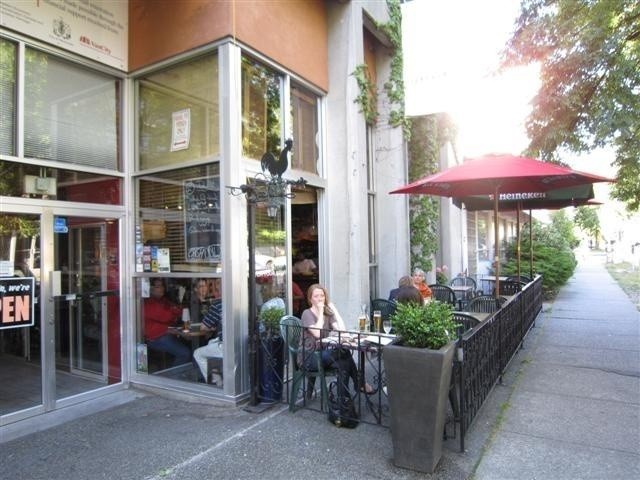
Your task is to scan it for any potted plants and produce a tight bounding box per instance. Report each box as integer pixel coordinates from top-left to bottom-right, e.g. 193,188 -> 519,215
383,296 -> 463,474
256,305 -> 288,402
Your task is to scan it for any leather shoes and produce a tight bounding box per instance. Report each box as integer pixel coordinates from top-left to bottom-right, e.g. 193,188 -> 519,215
354,384 -> 377,396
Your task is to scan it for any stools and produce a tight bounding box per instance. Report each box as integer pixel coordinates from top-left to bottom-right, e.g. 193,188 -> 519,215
147,344 -> 167,373
207,357 -> 224,388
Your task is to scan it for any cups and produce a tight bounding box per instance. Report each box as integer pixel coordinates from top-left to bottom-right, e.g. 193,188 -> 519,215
373,310 -> 381,331
383,320 -> 392,335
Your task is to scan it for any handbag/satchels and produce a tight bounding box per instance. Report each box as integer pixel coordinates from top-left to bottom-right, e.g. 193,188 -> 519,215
326,379 -> 361,430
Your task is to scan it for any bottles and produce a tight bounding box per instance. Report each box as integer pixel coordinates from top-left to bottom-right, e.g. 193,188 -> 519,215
358,303 -> 370,332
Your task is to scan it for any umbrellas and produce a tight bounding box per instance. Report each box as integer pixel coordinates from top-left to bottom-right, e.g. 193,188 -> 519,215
452,183 -> 595,290
500,199 -> 604,280
387,153 -> 620,298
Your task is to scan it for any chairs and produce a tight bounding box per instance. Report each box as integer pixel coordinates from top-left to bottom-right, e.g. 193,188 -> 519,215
371,275 -> 533,419
279,315 -> 335,413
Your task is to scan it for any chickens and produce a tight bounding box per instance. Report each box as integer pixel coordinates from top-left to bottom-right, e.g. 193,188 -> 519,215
259,138 -> 294,184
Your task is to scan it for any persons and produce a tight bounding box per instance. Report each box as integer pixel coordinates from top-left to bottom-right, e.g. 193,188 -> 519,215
192,278 -> 223,388
296,284 -> 380,418
387,275 -> 424,311
141,277 -> 192,381
180,277 -> 217,349
411,268 -> 433,306
254,246 -> 318,319
